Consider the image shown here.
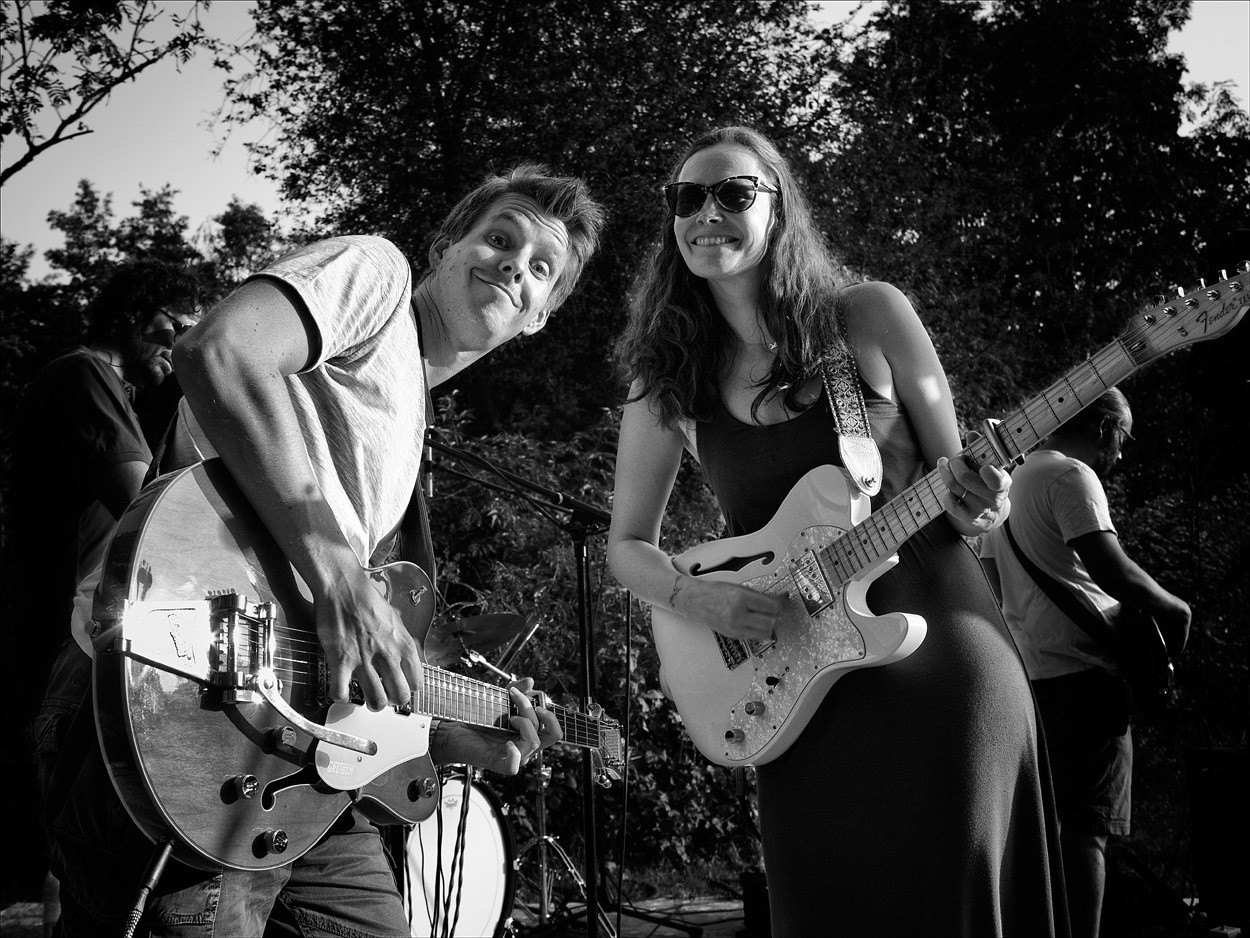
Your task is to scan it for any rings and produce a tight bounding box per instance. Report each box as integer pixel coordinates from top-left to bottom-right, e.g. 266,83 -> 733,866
960,488 -> 968,499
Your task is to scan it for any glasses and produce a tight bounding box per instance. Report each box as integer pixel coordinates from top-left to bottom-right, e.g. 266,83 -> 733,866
151,304 -> 193,343
661,175 -> 779,218
1107,415 -> 1136,452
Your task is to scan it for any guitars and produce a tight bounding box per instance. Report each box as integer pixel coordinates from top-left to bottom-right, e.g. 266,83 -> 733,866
1122,603 -> 1179,749
89,450 -> 629,880
649,252 -> 1250,777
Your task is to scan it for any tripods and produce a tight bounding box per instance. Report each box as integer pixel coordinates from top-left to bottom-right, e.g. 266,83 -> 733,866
434,440 -> 704,938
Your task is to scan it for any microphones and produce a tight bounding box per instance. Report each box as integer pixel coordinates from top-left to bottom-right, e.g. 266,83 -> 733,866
426,429 -> 433,497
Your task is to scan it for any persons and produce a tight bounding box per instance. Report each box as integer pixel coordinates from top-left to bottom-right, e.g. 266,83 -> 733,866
973,381 -> 1193,938
608,127 -> 1074,938
20,258 -> 210,938
82,160 -> 614,938
